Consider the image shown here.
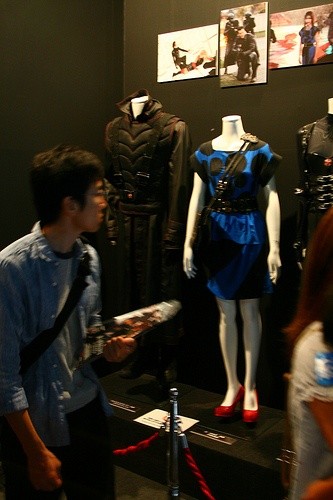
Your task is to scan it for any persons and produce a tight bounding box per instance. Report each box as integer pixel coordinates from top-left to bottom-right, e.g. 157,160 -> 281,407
0,146 -> 136,500
224,11 -> 261,83
284,97 -> 333,383
99,89 -> 191,389
299,6 -> 333,65
281,206 -> 333,500
269,20 -> 277,44
171,41 -> 190,70
182,115 -> 286,423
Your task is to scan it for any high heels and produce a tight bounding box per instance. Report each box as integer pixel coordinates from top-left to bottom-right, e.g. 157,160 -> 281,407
213,385 -> 245,418
242,390 -> 260,424
